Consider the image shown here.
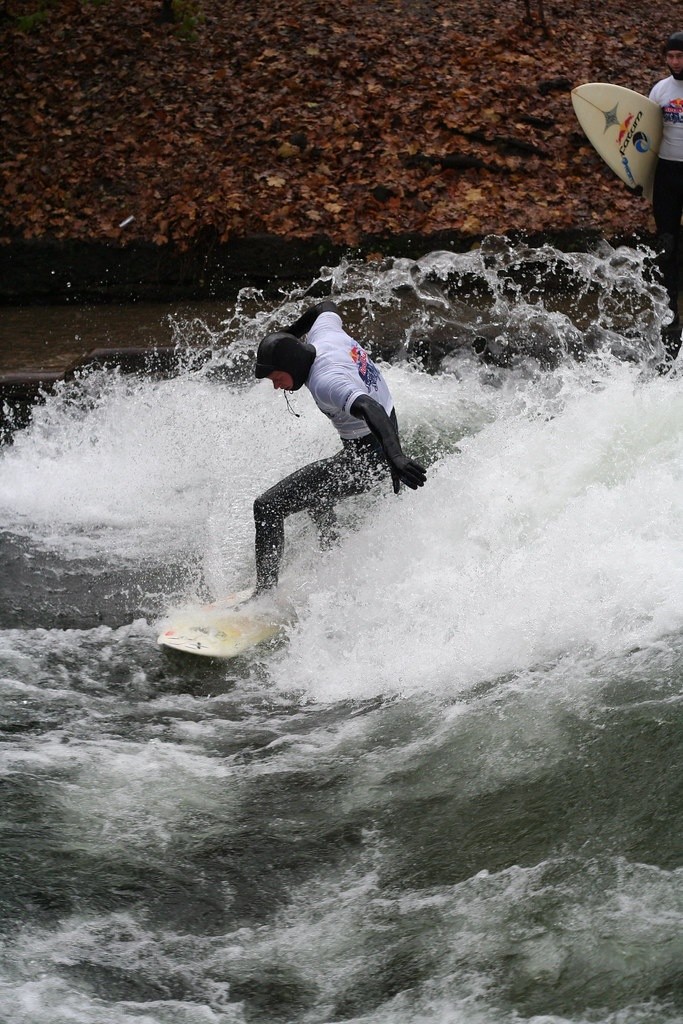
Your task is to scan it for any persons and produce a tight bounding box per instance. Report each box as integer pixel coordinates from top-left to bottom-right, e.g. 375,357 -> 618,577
242,301 -> 427,605
648,31 -> 683,379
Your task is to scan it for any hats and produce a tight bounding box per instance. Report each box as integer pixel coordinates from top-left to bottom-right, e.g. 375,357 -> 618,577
664,32 -> 683,80
255,334 -> 316,391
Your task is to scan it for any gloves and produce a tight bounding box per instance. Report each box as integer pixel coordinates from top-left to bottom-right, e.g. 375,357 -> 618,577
279,300 -> 340,338
624,182 -> 644,197
350,395 -> 427,495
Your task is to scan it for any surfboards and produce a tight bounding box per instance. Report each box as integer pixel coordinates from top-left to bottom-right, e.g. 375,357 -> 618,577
157,577 -> 295,660
570,82 -> 663,205
